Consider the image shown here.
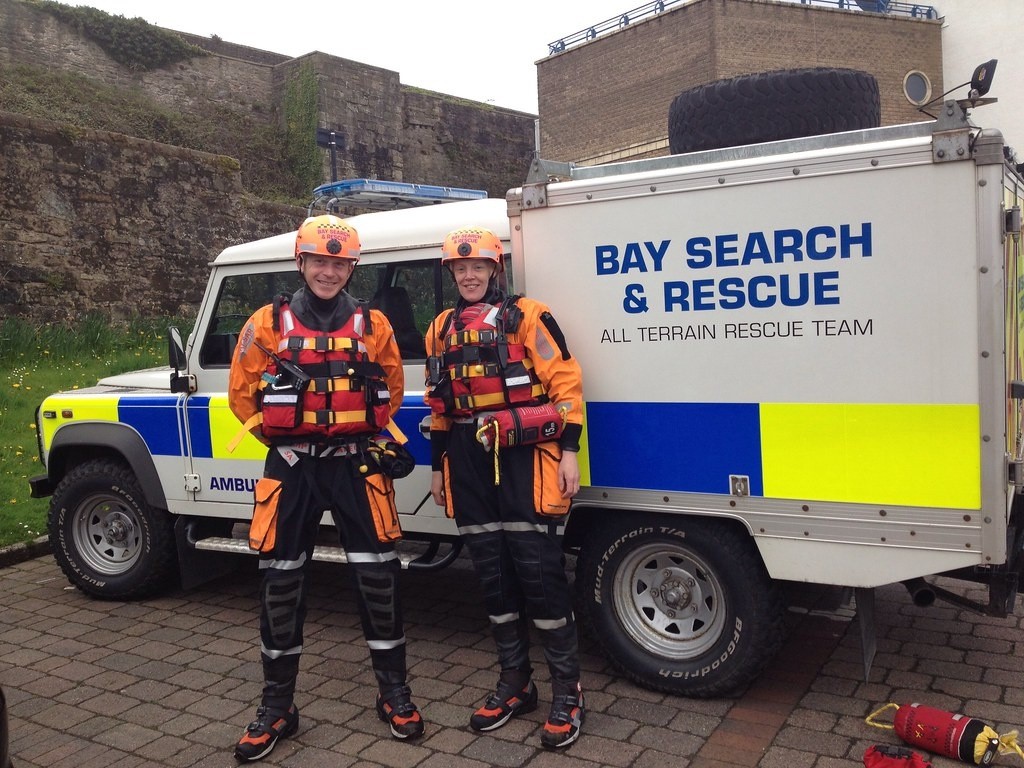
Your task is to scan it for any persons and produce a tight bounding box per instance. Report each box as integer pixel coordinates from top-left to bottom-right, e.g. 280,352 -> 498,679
229,214 -> 424,762
426,228 -> 584,748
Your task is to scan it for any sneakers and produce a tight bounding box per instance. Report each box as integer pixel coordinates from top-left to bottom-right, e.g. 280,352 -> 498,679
469,676 -> 539,731
540,680 -> 584,747
375,690 -> 425,739
235,702 -> 299,760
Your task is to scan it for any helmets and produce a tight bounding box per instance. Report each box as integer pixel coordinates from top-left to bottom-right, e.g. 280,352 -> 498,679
295,215 -> 361,264
441,229 -> 505,273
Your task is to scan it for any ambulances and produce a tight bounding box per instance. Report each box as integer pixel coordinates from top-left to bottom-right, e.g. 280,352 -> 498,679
24,58 -> 1024,702
